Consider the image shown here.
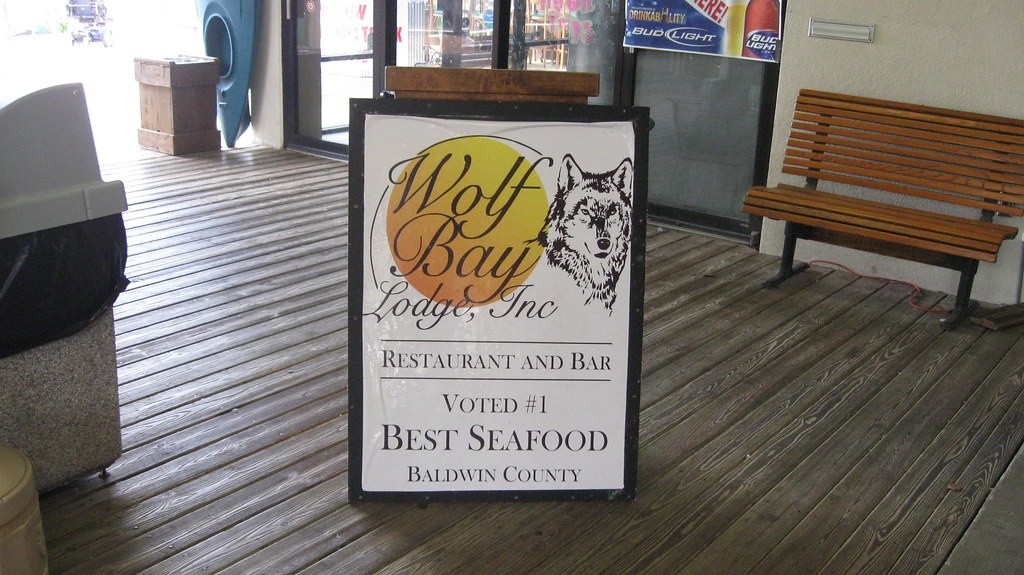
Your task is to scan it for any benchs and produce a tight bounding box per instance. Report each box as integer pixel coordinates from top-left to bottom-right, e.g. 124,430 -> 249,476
413,31 -> 540,67
741,90 -> 1024,330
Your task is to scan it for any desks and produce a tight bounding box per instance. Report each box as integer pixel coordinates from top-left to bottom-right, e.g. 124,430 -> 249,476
132,54 -> 221,155
384,64 -> 600,105
